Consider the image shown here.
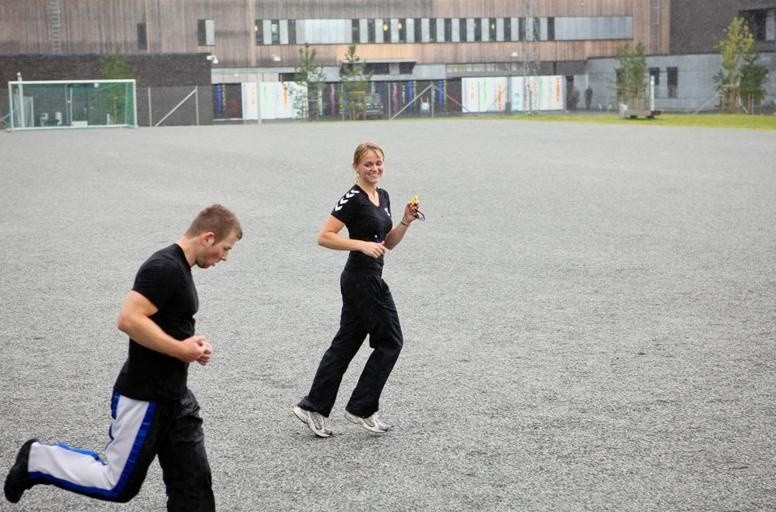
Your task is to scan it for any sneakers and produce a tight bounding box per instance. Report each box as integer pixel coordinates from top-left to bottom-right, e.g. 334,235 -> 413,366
344,411 -> 389,433
4,439 -> 38,503
294,407 -> 331,437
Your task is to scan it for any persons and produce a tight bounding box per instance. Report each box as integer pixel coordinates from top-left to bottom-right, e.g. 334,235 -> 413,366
568,86 -> 592,109
292,142 -> 420,437
4,204 -> 242,512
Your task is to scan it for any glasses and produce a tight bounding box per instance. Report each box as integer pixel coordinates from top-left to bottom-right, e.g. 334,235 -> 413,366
415,209 -> 425,221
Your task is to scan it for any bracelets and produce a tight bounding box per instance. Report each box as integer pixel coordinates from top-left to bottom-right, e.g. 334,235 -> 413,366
401,221 -> 409,227
